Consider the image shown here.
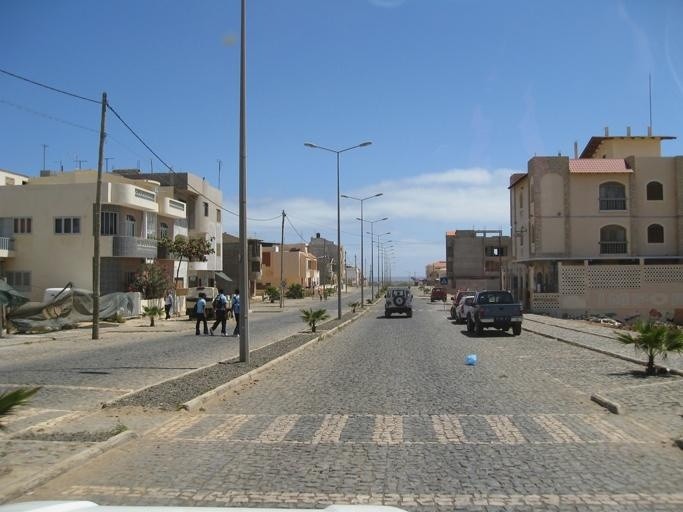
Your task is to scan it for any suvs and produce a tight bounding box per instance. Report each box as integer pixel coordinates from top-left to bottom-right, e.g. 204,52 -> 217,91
383,286 -> 414,320
448,287 -> 527,337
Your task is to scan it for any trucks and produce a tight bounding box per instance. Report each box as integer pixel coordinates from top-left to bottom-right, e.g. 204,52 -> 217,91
184,285 -> 234,322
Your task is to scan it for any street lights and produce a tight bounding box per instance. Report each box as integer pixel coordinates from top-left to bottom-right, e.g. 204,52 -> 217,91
351,213 -> 399,294
339,190 -> 385,311
302,140 -> 373,323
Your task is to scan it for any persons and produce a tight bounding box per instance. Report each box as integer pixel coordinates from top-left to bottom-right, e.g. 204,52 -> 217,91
209,288 -> 228,336
195,292 -> 207,335
230,289 -> 240,337
163,292 -> 173,319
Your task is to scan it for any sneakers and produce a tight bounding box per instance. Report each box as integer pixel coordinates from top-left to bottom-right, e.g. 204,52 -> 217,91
165,315 -> 171,320
196,328 -> 240,337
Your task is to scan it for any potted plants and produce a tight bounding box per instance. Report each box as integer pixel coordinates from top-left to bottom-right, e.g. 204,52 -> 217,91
299,305 -> 329,332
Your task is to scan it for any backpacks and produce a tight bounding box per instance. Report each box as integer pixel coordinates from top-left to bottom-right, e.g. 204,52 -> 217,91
233,296 -> 240,313
212,294 -> 225,312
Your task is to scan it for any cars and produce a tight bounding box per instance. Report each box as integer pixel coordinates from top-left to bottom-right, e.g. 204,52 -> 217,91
430,287 -> 449,303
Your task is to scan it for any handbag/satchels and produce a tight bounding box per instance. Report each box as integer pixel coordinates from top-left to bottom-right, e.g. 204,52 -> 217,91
194,303 -> 198,314
